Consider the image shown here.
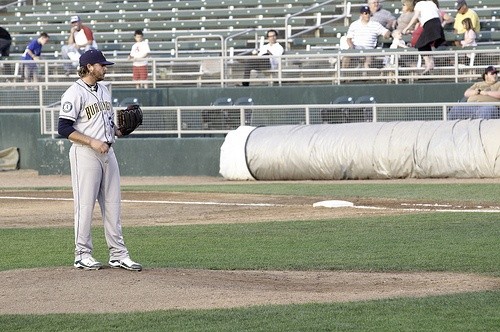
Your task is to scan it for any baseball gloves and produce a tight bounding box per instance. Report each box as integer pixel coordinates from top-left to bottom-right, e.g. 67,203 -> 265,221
115,103 -> 143,137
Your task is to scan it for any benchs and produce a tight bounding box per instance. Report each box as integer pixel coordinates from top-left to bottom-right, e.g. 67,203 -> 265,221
0,0 -> 500,91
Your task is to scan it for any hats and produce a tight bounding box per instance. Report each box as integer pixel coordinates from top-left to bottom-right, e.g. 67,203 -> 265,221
455,0 -> 466,10
71,15 -> 80,24
486,66 -> 499,74
360,6 -> 370,14
79,50 -> 114,66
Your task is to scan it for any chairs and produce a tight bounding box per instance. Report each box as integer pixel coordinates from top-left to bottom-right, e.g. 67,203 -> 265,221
321,96 -> 376,124
203,97 -> 253,137
112,98 -> 147,138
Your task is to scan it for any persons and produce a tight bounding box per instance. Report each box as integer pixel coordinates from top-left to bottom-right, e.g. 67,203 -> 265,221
446,0 -> 480,65
237,30 -> 284,86
392,0 -> 418,36
454,18 -> 478,81
446,66 -> 500,120
21,33 -> 48,90
58,50 -> 143,271
338,0 -> 397,61
401,0 -> 445,80
380,30 -> 410,82
411,0 -> 455,48
67,16 -> 98,76
128,30 -> 150,88
335,6 -> 392,84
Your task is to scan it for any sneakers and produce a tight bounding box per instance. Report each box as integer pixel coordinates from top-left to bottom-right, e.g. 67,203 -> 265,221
108,255 -> 142,272
74,256 -> 102,271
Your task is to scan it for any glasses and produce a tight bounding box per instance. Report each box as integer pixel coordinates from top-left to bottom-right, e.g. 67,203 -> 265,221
364,12 -> 370,15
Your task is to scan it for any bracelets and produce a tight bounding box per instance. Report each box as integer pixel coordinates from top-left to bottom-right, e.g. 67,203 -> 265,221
477,89 -> 481,94
